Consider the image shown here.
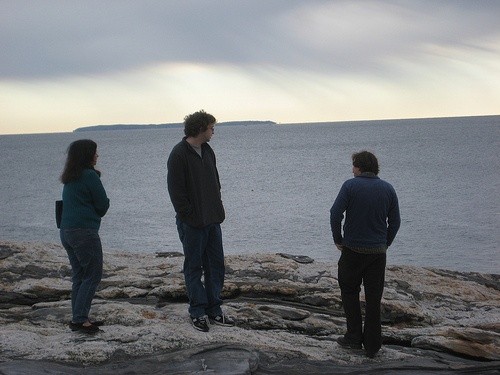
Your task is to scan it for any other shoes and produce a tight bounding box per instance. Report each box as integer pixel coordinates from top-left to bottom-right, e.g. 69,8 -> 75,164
365,347 -> 379,358
337,335 -> 363,350
68,319 -> 105,334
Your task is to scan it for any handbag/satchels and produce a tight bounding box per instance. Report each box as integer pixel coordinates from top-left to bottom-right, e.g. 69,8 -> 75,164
55,200 -> 63,228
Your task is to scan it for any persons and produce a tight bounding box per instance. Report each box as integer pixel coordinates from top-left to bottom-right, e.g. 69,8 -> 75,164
329,152 -> 401,359
166,110 -> 237,332
60,138 -> 110,333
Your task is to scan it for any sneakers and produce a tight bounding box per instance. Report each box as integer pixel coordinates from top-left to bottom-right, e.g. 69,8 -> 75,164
211,312 -> 235,327
191,312 -> 209,332
